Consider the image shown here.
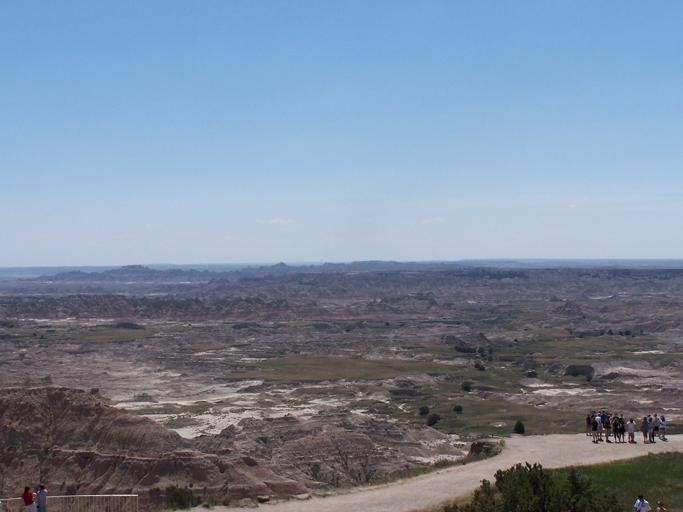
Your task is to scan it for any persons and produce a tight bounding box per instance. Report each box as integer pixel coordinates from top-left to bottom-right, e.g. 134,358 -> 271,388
586,410 -> 667,444
21,483 -> 48,512
633,494 -> 668,512
